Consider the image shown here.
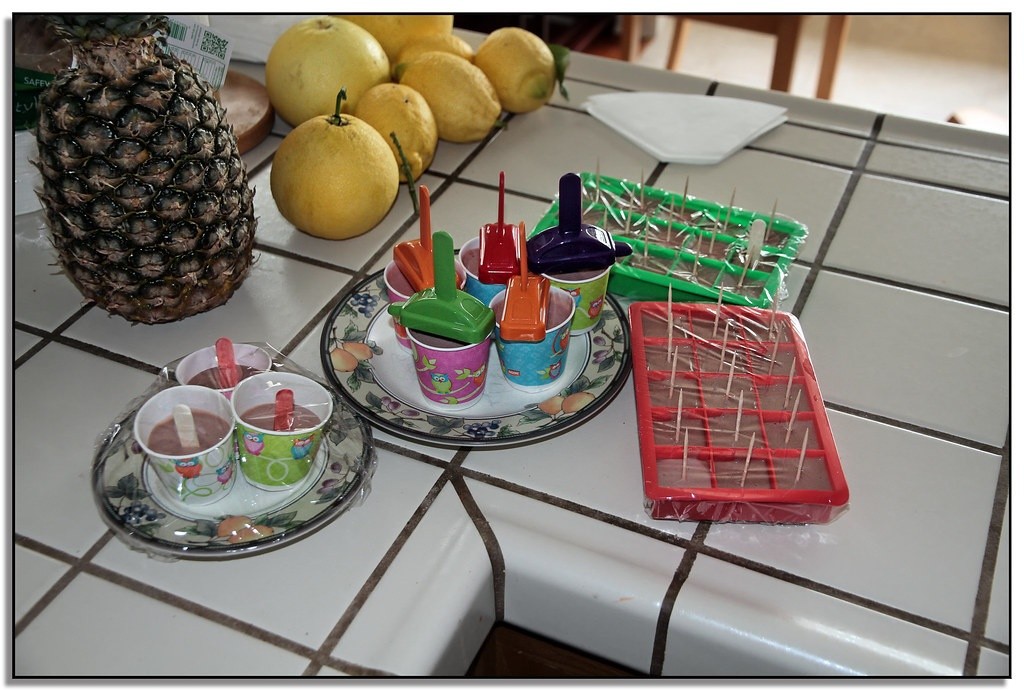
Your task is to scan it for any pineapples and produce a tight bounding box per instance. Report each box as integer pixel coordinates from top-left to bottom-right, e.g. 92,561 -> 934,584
25,13 -> 261,324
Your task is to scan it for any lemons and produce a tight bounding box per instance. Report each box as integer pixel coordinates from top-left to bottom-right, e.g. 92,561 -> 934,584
355,27 -> 556,183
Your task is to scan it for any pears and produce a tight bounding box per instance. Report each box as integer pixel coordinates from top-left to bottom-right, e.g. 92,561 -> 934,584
270,114 -> 399,241
326,15 -> 453,60
264,17 -> 390,128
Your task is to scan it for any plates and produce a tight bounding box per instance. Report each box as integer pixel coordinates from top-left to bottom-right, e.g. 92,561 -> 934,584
320,267 -> 633,450
90,370 -> 374,560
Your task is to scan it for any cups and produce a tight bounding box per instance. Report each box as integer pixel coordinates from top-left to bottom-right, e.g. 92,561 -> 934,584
134,384 -> 236,504
489,285 -> 576,394
230,372 -> 332,491
405,323 -> 496,413
541,267 -> 611,336
459,236 -> 508,307
175,343 -> 273,401
384,257 -> 467,355
